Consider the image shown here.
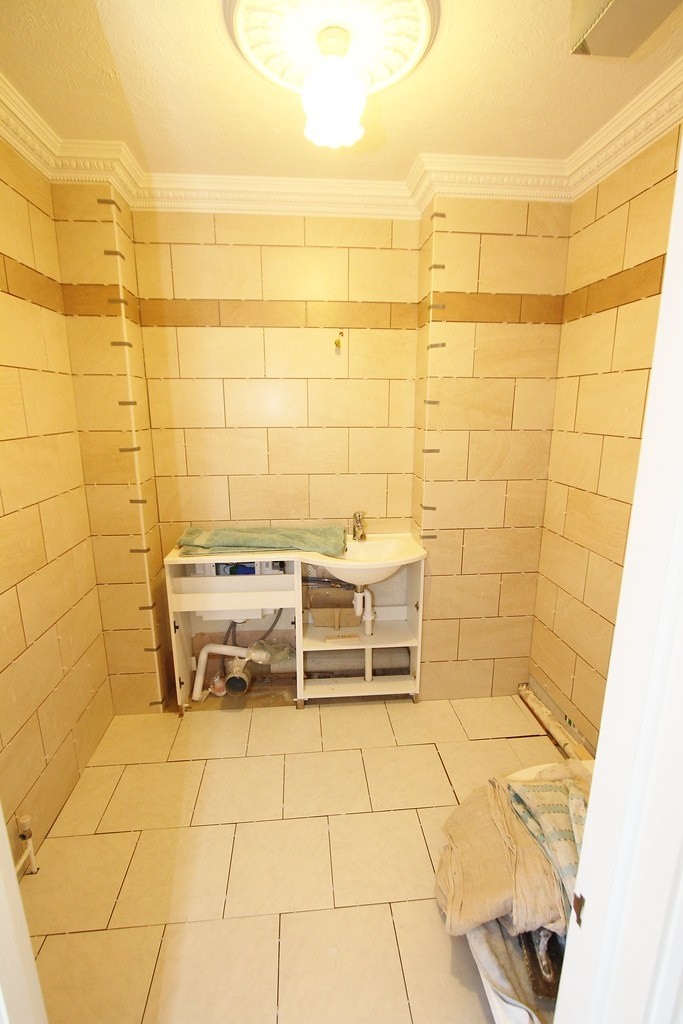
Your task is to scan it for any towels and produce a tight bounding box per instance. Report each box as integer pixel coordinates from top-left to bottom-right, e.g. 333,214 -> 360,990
175,525 -> 348,558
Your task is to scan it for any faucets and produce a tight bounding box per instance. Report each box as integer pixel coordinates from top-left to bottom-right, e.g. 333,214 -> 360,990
352,511 -> 366,542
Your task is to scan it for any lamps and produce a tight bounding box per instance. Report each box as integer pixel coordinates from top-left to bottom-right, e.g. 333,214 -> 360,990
222,0 -> 441,150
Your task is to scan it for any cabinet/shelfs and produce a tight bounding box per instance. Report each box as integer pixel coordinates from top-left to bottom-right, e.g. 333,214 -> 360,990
163,532 -> 429,716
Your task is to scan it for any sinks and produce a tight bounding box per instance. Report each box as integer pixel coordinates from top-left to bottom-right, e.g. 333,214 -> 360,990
319,539 -> 400,564
